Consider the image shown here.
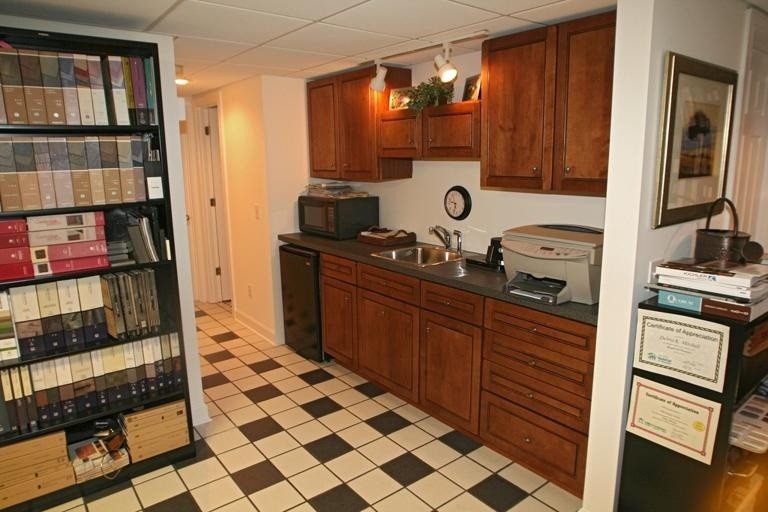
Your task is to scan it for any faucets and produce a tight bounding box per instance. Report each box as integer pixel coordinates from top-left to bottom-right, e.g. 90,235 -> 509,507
453,230 -> 462,254
428,225 -> 451,250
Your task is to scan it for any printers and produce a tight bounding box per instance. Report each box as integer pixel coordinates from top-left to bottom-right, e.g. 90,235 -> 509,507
499,222 -> 603,308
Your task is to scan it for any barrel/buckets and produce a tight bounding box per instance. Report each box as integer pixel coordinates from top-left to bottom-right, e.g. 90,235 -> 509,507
694,197 -> 751,266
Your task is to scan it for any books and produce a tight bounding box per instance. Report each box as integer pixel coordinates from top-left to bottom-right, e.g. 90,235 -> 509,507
644,258 -> 768,358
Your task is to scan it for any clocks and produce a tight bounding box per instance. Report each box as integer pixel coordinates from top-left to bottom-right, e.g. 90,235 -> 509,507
444,186 -> 472,221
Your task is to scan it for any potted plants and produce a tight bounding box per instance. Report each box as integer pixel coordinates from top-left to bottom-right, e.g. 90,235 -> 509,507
407,77 -> 455,115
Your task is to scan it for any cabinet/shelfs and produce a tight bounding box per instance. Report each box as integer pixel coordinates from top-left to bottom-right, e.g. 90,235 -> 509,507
477,295 -> 596,500
320,250 -> 419,409
1,23 -> 195,512
419,279 -> 484,438
379,101 -> 480,162
613,296 -> 768,512
479,10 -> 620,199
306,66 -> 412,183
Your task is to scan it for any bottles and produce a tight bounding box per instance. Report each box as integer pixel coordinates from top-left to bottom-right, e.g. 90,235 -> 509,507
486,236 -> 505,273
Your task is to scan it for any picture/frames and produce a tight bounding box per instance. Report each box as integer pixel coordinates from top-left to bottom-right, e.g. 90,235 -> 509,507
462,74 -> 481,101
652,51 -> 739,233
388,87 -> 415,109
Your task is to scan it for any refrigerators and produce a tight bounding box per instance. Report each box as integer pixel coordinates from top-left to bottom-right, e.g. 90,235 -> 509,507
279,244 -> 321,363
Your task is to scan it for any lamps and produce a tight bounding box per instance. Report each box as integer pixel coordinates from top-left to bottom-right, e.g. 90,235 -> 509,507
175,64 -> 190,87
369,41 -> 459,92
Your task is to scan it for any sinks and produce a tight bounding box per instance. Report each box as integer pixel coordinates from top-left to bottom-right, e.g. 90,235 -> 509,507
370,244 -> 465,268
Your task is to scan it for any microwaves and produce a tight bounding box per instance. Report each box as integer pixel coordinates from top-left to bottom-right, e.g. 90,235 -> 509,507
297,193 -> 379,240
